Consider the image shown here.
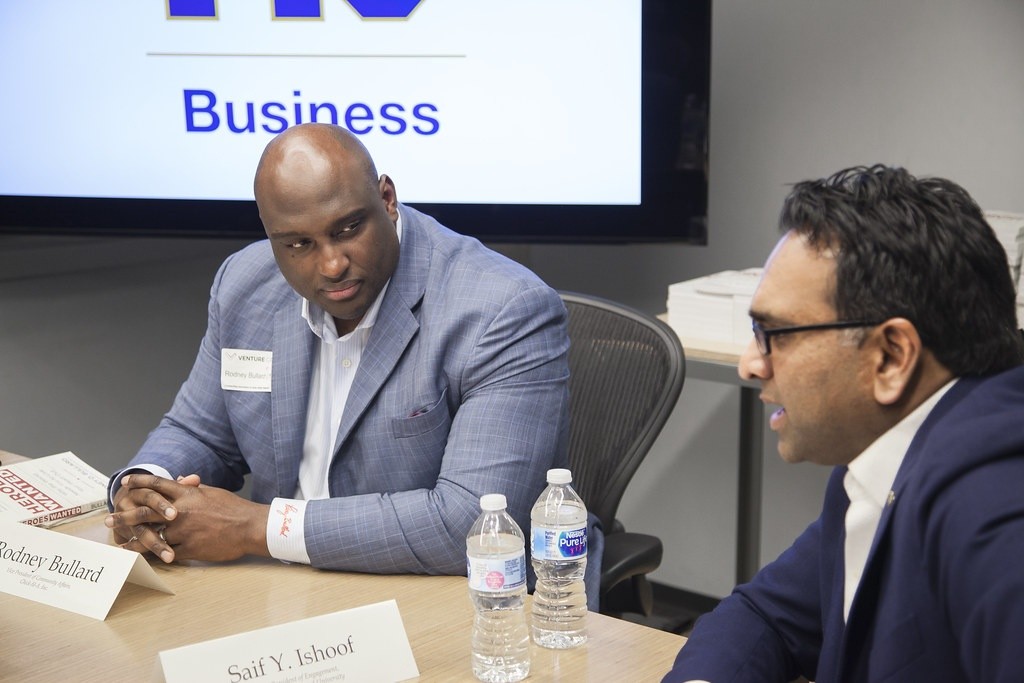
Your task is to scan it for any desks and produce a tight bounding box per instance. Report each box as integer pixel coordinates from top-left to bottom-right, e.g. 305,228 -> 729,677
615,313 -> 763,601
0,451 -> 689,683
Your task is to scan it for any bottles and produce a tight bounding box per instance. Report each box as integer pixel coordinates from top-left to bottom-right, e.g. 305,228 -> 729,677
530,468 -> 588,649
466,493 -> 531,683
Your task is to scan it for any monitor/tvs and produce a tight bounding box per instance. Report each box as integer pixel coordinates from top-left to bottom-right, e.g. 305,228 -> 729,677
0,0 -> 713,248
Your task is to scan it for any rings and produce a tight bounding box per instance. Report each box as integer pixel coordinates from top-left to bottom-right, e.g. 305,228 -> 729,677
157,528 -> 168,543
127,535 -> 138,543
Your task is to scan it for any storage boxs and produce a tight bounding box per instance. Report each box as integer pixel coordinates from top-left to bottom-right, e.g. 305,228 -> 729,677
667,267 -> 764,360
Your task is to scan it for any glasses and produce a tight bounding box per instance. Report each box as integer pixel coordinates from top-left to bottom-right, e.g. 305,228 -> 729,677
750,319 -> 883,356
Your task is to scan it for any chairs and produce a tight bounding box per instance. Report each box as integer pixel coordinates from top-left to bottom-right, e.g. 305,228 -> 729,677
551,290 -> 685,616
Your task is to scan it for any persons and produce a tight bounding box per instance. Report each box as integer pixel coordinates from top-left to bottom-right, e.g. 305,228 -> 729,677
104,123 -> 604,617
659,166 -> 1024,681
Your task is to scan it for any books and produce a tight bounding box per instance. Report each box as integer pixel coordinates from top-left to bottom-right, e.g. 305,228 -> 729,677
1,450 -> 112,524
985,209 -> 1024,330
665,267 -> 763,358
